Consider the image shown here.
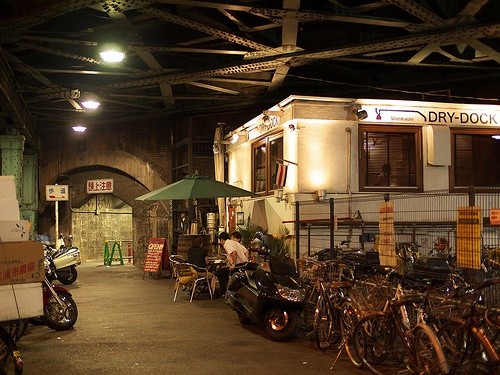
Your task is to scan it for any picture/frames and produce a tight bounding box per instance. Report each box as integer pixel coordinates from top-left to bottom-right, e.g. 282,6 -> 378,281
236,212 -> 244,225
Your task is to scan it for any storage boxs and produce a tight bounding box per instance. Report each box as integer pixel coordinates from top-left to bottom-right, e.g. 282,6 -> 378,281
0,176 -> 46,320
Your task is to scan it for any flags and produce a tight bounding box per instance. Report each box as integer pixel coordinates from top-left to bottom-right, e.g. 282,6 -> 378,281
273,162 -> 287,186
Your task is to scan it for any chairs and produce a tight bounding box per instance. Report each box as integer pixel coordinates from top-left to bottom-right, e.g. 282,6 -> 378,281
169,254 -> 213,303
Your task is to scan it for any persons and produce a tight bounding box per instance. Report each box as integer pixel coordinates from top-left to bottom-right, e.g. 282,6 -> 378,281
187,237 -> 209,273
214,232 -> 249,293
230,231 -> 241,243
433,238 -> 448,254
378,163 -> 390,185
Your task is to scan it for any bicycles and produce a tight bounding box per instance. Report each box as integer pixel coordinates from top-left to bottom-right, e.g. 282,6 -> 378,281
304,248 -> 500,375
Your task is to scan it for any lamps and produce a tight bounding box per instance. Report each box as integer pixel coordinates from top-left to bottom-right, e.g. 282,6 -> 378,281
99,53 -> 127,62
81,102 -> 100,108
263,115 -> 270,124
72,127 -> 87,132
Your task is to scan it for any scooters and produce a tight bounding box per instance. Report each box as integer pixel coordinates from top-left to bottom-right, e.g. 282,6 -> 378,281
1,242 -> 81,375
225,233 -> 305,342
